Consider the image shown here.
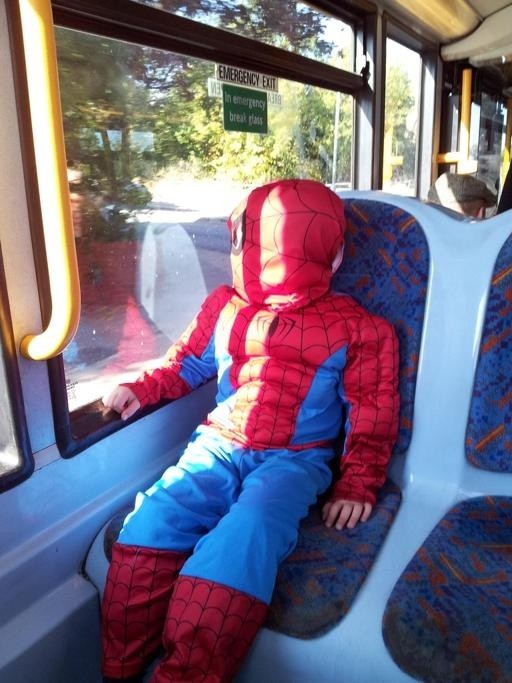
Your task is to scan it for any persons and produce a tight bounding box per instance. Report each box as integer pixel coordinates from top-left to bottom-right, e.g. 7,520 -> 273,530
64,152 -> 160,386
100,179 -> 402,683
425,171 -> 497,221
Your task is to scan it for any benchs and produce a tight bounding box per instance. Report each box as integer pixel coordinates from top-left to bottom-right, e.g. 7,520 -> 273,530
85,189 -> 512,683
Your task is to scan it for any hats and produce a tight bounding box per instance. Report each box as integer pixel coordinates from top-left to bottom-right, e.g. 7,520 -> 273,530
426,172 -> 498,208
